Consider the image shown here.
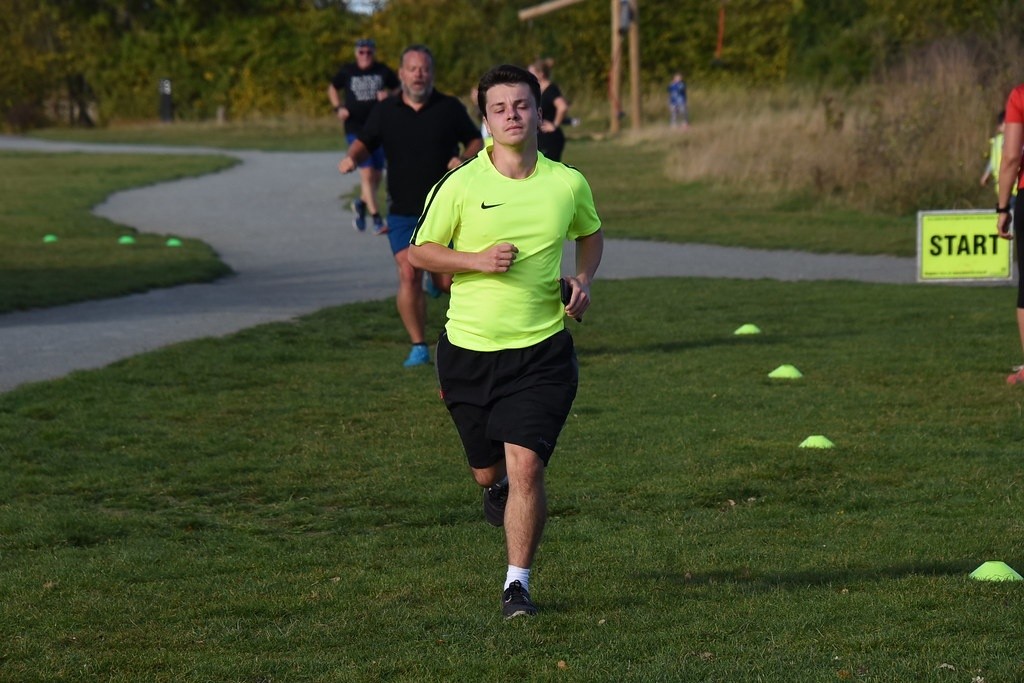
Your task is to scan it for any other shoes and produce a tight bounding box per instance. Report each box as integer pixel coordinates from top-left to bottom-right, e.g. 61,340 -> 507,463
425,270 -> 441,299
351,198 -> 366,232
1007,365 -> 1024,385
374,221 -> 389,235
403,344 -> 430,368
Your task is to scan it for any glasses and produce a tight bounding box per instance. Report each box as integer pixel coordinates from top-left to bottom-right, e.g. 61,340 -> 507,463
359,50 -> 373,55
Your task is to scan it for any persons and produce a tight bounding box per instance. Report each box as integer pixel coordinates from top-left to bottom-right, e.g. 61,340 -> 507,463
666,74 -> 688,130
980,82 -> 1024,387
326,37 -> 602,622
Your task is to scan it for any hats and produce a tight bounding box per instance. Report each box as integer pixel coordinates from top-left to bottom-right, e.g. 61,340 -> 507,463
358,39 -> 375,47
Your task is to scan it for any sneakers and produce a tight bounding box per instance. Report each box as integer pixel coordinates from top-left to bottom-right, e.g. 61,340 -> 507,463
483,475 -> 508,528
502,579 -> 538,620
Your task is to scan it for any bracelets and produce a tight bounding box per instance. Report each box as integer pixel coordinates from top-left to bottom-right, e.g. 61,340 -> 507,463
996,203 -> 1010,213
